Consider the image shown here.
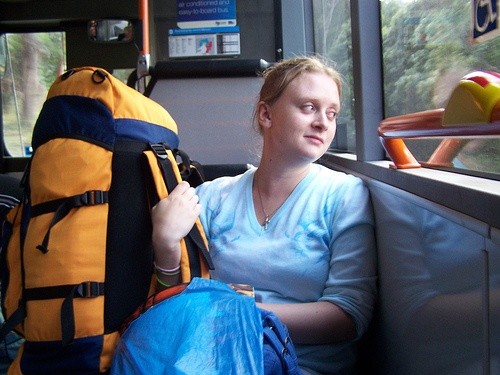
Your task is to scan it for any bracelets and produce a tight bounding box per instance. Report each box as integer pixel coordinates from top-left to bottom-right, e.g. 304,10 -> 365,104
153,262 -> 180,276
156,277 -> 170,290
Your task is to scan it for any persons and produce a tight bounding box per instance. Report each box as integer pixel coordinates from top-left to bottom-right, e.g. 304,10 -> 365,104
367,58 -> 500,375
151,57 -> 377,375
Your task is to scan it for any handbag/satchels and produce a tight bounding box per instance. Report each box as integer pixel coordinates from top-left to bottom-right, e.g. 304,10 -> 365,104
111,277 -> 304,375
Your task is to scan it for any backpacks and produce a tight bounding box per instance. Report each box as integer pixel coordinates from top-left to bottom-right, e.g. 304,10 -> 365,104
0,67 -> 215,375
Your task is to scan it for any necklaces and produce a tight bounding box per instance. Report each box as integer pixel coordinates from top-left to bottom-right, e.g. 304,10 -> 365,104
257,175 -> 270,230
